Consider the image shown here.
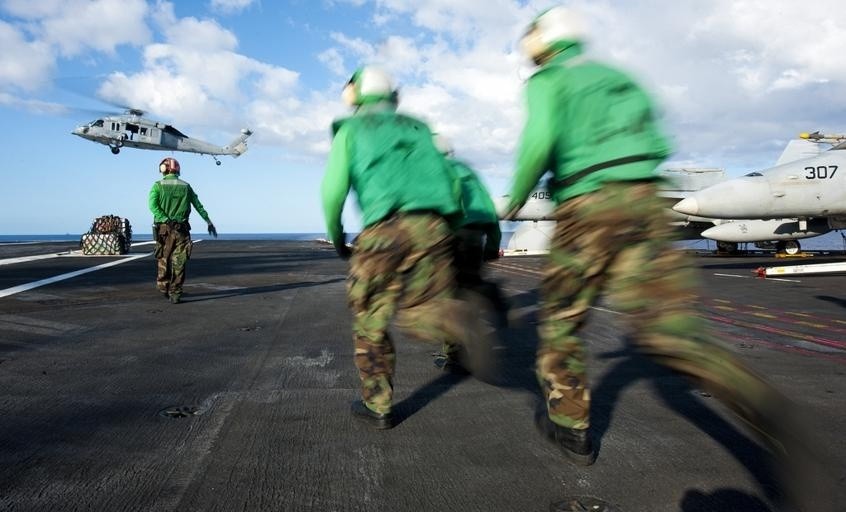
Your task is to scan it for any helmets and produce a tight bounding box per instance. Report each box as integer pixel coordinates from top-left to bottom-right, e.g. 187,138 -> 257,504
519,8 -> 586,62
341,66 -> 398,107
158,158 -> 180,174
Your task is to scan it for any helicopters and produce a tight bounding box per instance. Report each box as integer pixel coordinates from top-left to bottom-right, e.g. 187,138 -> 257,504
54,82 -> 255,170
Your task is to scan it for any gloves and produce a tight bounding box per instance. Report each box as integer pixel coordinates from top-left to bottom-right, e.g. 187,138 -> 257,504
207,223 -> 218,236
171,222 -> 188,235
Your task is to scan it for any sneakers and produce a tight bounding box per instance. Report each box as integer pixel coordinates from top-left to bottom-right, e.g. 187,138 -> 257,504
350,399 -> 393,429
534,404 -> 596,466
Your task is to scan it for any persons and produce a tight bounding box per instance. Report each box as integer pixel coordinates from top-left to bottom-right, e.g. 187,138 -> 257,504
430,137 -> 508,382
321,61 -> 504,427
148,158 -> 217,304
499,4 -> 832,511
